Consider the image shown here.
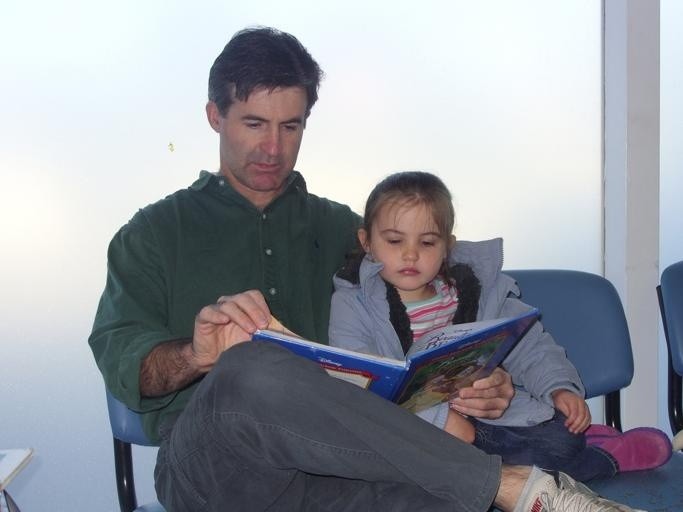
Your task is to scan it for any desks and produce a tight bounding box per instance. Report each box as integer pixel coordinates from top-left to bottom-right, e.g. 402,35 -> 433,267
0,448 -> 34,493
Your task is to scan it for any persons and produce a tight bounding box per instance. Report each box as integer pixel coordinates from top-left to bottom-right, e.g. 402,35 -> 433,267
85,20 -> 646,512
326,169 -> 673,482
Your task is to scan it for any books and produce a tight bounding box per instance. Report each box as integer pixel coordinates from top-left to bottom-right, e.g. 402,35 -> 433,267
251,307 -> 544,414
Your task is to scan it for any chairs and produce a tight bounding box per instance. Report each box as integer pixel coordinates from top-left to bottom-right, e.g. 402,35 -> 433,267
106,385 -> 159,512
500,269 -> 683,512
656,261 -> 683,454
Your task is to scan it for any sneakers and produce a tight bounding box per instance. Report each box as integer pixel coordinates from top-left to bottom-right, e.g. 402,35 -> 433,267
583,425 -> 622,437
585,427 -> 673,472
522,467 -> 648,512
